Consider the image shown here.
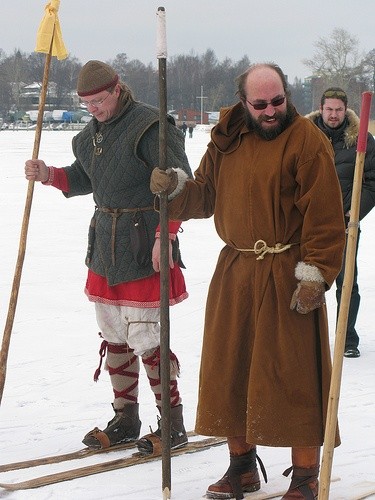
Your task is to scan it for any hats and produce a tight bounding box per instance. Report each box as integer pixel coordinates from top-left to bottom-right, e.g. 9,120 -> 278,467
77,60 -> 118,96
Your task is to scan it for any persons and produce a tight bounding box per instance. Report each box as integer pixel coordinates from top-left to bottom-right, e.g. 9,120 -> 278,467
25,62 -> 194,455
182,122 -> 194,139
149,64 -> 346,500
305,85 -> 375,358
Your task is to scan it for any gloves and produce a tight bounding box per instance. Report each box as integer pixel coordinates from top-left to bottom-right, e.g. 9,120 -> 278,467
150,167 -> 187,200
289,262 -> 326,314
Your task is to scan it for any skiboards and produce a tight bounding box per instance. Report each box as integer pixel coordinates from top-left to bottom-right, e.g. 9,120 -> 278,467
0,430 -> 227,490
227,475 -> 375,500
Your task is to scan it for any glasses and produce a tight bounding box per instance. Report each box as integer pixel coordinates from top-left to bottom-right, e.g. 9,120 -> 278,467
246,90 -> 287,110
323,91 -> 346,97
81,87 -> 114,108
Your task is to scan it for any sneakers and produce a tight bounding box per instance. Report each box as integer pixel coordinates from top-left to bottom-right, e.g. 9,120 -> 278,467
344,347 -> 360,357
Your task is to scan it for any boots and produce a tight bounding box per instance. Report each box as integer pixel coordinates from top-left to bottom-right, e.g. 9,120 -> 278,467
281,446 -> 320,500
82,403 -> 141,450
206,436 -> 260,498
135,404 -> 188,454
